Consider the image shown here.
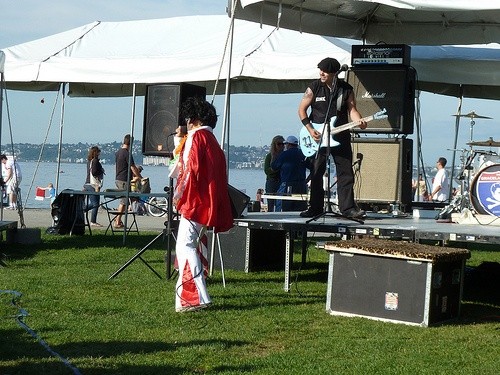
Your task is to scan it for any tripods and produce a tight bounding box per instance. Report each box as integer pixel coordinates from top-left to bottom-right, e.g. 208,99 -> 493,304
433,120 -> 477,220
106,162 -> 178,281
306,75 -> 364,224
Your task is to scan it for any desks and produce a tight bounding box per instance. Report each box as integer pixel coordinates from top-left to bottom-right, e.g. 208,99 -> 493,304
62,190 -> 174,237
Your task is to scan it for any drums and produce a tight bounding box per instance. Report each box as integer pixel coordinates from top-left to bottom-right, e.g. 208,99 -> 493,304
477,150 -> 499,168
470,163 -> 500,217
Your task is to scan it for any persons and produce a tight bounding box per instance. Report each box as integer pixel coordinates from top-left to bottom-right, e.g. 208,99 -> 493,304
84,146 -> 107,226
115,133 -> 149,228
413,173 -> 427,201
168,99 -> 234,313
264,135 -> 287,212
1,154 -> 22,210
271,136 -> 308,212
37,182 -> 58,211
299,57 -> 369,219
426,157 -> 450,203
256,188 -> 264,205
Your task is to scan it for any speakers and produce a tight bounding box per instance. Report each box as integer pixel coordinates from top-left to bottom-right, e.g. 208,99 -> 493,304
348,137 -> 414,207
141,82 -> 205,158
344,66 -> 415,133
228,183 -> 250,217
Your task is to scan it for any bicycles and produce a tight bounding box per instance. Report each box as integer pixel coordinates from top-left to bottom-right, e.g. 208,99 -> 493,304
100,177 -> 168,217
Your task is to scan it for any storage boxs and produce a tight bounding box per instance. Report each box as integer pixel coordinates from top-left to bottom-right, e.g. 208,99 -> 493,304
327,250 -> 467,328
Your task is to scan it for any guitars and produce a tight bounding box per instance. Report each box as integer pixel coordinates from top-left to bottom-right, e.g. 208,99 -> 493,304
300,107 -> 388,158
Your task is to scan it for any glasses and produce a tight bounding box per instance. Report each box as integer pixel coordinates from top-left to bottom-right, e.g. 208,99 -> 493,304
276,142 -> 284,145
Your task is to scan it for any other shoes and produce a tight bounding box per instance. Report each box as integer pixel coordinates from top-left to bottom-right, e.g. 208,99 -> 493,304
114,222 -> 125,228
300,208 -> 323,217
344,208 -> 366,219
181,303 -> 212,310
90,222 -> 103,226
5,205 -> 17,210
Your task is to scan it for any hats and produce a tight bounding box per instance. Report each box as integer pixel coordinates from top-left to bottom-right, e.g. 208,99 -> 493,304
283,136 -> 298,145
317,58 -> 340,73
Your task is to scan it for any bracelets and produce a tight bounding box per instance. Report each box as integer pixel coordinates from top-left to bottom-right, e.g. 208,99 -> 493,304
302,117 -> 310,126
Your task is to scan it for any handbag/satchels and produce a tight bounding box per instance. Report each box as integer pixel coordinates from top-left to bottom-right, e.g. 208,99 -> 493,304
89,174 -> 104,188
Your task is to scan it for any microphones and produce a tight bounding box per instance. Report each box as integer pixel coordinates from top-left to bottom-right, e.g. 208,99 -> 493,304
170,124 -> 187,136
334,64 -> 349,77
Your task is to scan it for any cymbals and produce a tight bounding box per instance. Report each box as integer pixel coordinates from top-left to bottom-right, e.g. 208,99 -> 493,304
453,113 -> 493,120
466,138 -> 500,147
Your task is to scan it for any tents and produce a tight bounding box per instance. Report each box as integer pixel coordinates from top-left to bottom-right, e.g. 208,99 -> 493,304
0,15 -> 500,98
227,0 -> 500,46
